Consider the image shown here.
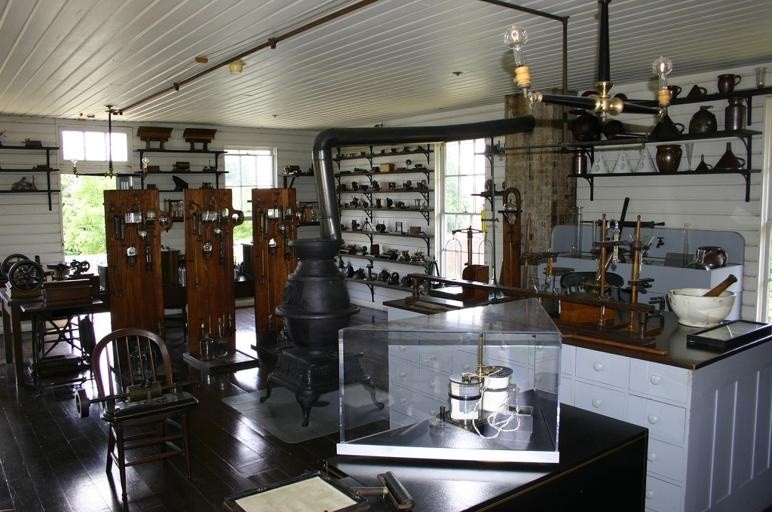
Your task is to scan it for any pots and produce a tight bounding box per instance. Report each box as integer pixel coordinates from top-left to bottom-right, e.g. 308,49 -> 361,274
695,247 -> 727,270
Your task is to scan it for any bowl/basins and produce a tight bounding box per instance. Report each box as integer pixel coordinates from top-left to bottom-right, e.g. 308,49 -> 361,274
668,288 -> 736,328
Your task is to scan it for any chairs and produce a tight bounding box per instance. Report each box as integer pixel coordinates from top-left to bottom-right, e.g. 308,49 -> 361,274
89,327 -> 200,512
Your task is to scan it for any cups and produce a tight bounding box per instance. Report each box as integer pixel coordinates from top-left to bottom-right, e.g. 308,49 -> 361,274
668,74 -> 742,101
755,66 -> 767,88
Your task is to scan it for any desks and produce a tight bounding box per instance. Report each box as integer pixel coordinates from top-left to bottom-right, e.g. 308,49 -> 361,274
219,394 -> 649,511
181,346 -> 260,397
0,283 -> 114,404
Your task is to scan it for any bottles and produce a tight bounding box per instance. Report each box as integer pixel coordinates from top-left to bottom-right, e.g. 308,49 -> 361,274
689,98 -> 747,134
656,145 -> 682,174
574,115 -> 602,141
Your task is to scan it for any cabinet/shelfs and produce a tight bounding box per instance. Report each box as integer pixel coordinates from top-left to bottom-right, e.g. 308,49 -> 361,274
382,290 -> 772,511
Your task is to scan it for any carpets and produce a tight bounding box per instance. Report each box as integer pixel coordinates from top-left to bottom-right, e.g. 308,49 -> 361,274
221,369 -> 389,444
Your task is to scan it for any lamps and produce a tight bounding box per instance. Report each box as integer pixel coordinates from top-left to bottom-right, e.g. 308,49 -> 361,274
70,157 -> 79,168
504,24 -> 535,98
142,156 -> 150,169
651,55 -> 674,106
